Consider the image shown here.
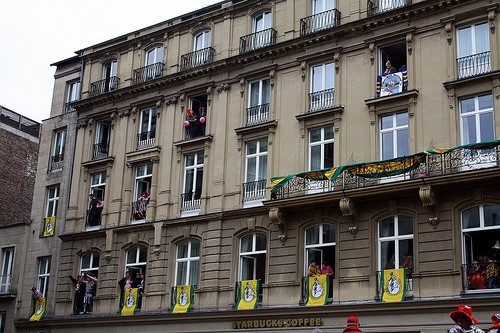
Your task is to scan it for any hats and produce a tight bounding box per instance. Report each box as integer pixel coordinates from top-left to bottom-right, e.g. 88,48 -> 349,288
347,316 -> 359,325
450,304 -> 478,326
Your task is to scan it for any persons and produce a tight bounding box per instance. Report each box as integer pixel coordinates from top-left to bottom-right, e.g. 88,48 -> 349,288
88,194 -> 104,208
308,259 -> 333,276
487,314 -> 500,333
118,271 -> 145,310
449,304 -> 485,333
68,271 -> 97,314
382,61 -> 396,76
138,192 -> 149,212
472,256 -> 499,289
401,251 -> 413,267
186,110 -> 197,122
343,316 -> 362,333
31,287 -> 43,298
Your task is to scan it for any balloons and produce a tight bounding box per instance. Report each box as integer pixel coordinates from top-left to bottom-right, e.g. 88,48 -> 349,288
200,117 -> 205,123
184,121 -> 189,125
